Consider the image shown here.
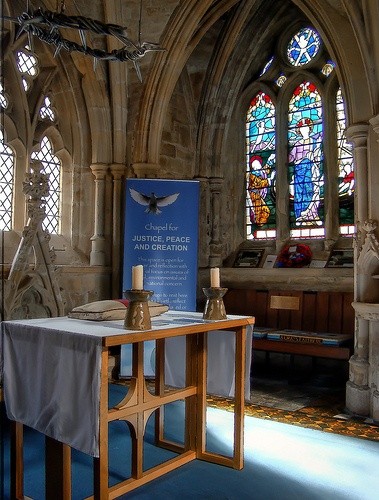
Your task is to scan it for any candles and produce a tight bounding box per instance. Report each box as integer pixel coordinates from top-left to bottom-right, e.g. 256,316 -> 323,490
132,264 -> 143,289
210,267 -> 220,286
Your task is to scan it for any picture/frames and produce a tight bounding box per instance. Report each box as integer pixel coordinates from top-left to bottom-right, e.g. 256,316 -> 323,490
325,248 -> 354,268
232,248 -> 265,268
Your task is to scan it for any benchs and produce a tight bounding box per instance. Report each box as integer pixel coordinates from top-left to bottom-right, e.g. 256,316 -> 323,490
252,327 -> 351,391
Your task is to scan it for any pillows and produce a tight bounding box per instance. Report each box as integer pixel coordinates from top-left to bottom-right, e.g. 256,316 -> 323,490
68,299 -> 170,320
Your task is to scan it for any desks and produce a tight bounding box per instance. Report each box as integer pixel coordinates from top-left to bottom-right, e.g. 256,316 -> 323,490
0,310 -> 258,500
